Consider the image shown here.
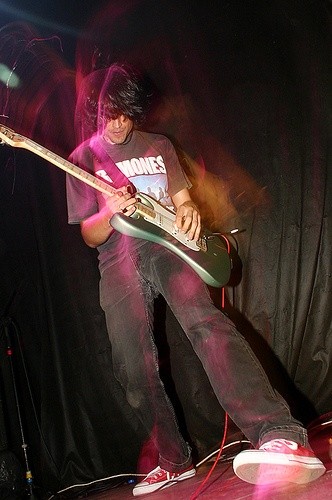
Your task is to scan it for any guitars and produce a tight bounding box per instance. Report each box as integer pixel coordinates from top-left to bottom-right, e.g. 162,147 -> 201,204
0,124 -> 234,287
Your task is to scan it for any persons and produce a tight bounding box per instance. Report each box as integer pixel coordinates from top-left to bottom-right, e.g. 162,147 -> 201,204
66,64 -> 327,496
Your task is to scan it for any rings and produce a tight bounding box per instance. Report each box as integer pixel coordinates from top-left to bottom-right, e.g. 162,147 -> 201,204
117,191 -> 123,197
119,204 -> 124,210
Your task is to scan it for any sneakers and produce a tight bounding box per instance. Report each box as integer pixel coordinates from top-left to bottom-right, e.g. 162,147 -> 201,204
132,462 -> 197,496
233,437 -> 326,485
